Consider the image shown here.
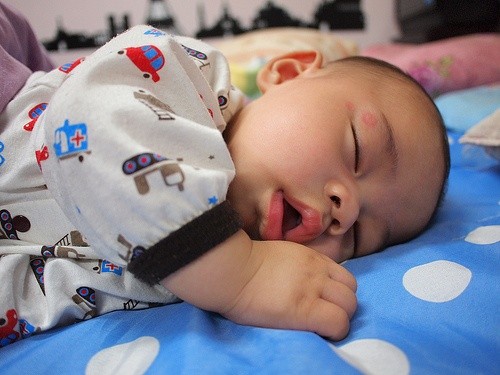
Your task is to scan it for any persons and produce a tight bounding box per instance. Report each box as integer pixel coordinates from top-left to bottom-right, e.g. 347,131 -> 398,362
0,25 -> 452,347
